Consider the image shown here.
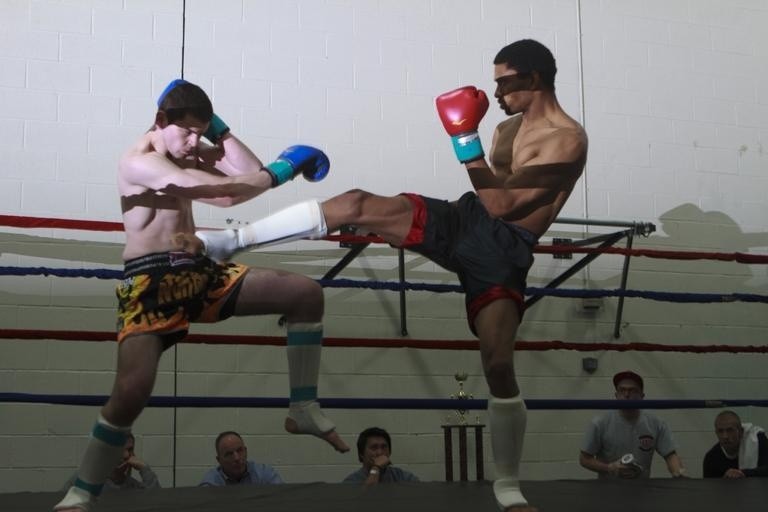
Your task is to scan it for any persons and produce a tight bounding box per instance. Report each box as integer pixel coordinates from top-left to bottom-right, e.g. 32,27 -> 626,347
60,432 -> 162,492
578,369 -> 690,479
703,410 -> 768,479
341,427 -> 421,483
169,40 -> 589,512
199,432 -> 284,486
51,80 -> 351,512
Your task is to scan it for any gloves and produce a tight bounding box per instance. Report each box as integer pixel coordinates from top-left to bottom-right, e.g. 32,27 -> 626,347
260,145 -> 329,188
157,79 -> 229,145
436,85 -> 490,164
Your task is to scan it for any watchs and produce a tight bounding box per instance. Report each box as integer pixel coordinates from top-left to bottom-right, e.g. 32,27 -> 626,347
369,469 -> 381,475
